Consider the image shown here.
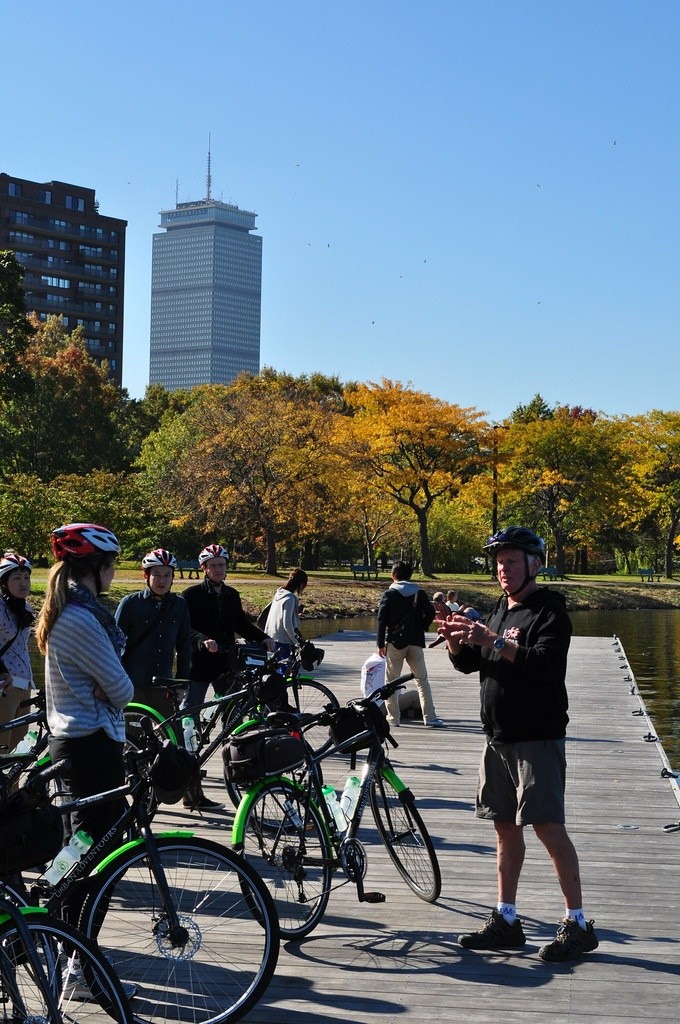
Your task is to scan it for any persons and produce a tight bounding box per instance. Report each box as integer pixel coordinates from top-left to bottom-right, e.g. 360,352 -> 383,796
0,552 -> 33,755
264,568 -> 308,661
433,589 -> 485,619
114,545 -> 274,811
31,520 -> 135,999
361,560 -> 444,727
438,525 -> 598,963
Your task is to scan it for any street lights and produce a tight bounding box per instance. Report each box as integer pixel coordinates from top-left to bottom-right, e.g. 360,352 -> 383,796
493,425 -> 510,582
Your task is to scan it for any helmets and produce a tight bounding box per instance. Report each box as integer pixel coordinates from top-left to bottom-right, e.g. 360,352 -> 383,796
483,526 -> 547,566
142,549 -> 178,570
198,544 -> 229,566
0,554 -> 32,578
52,523 -> 121,561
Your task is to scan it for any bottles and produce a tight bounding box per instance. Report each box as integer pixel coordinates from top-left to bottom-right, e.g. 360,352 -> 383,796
44,831 -> 93,886
182,717 -> 197,752
340,776 -> 361,822
321,784 -> 347,833
202,693 -> 222,720
2,730 -> 39,773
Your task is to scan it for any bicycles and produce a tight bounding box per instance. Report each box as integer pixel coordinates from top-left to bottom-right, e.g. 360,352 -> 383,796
221,673 -> 442,940
0,627 -> 341,1024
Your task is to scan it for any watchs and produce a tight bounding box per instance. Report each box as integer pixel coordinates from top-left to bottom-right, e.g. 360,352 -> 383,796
489,637 -> 505,660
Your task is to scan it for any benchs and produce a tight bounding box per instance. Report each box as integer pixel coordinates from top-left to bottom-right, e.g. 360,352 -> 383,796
175,560 -> 203,580
638,568 -> 663,582
350,563 -> 379,581
542,567 -> 565,581
428,600 -> 464,648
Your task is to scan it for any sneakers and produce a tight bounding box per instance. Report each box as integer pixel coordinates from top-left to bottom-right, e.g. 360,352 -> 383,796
459,912 -> 527,950
539,917 -> 600,959
182,795 -> 223,810
67,952 -> 114,976
61,967 -> 137,1003
426,719 -> 444,727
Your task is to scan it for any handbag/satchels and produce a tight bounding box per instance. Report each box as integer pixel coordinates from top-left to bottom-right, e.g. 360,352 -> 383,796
389,611 -> 416,650
361,655 -> 386,706
256,602 -> 271,630
